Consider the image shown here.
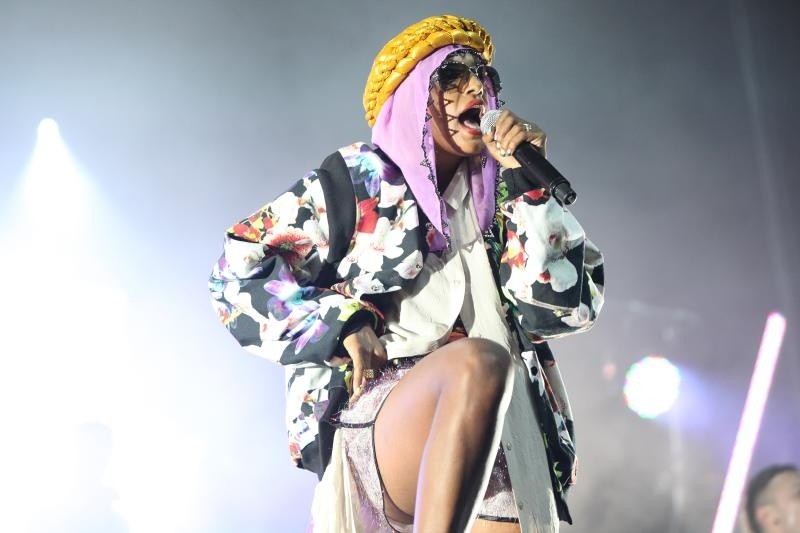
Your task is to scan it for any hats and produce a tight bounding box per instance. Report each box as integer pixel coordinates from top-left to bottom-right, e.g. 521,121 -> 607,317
362,16 -> 495,128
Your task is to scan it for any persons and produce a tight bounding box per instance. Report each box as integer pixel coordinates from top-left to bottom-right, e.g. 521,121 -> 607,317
208,15 -> 604,533
746,465 -> 800,533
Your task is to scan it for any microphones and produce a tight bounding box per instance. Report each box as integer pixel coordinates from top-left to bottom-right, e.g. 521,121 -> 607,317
479,108 -> 578,207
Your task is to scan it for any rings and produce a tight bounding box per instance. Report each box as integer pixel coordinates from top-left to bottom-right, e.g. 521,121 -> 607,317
364,369 -> 378,378
523,124 -> 532,131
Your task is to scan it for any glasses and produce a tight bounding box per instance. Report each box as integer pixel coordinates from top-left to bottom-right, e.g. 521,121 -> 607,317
429,62 -> 503,98
